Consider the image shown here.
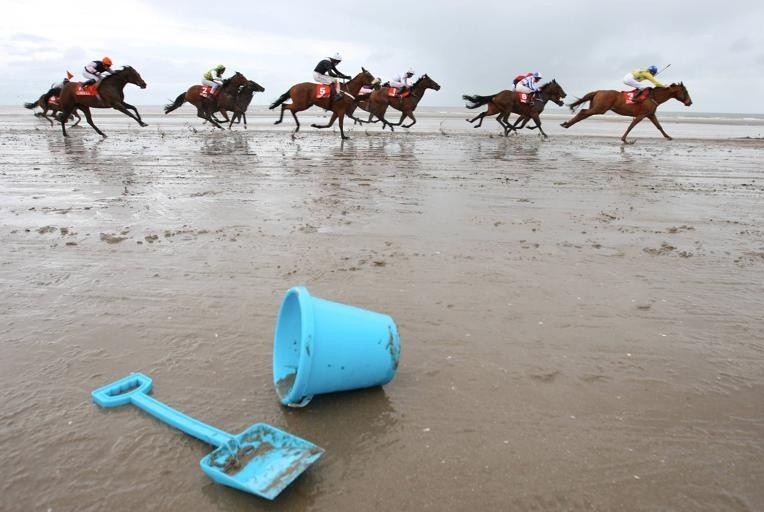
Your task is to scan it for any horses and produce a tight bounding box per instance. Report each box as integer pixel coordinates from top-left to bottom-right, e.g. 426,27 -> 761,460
559,82 -> 692,143
268,67 -> 377,140
163,70 -> 264,130
355,73 -> 441,131
462,78 -> 565,141
25,66 -> 149,138
344,80 -> 391,124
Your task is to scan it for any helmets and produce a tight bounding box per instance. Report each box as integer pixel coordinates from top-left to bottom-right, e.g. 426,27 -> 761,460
216,64 -> 225,72
330,52 -> 342,61
648,66 -> 658,74
530,71 -> 542,79
406,67 -> 414,75
102,57 -> 112,66
64,78 -> 69,83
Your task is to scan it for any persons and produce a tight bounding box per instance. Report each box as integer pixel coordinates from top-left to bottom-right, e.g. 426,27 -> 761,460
201,64 -> 226,102
78,57 -> 114,92
515,71 -> 546,109
511,72 -> 534,88
388,68 -> 418,101
358,77 -> 383,103
621,64 -> 669,103
310,52 -> 352,104
46,78 -> 69,104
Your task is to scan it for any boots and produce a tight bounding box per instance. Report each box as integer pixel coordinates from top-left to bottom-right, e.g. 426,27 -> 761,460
526,92 -> 535,105
395,85 -> 406,99
330,83 -> 343,103
631,90 -> 643,103
79,78 -> 96,88
206,93 -> 216,102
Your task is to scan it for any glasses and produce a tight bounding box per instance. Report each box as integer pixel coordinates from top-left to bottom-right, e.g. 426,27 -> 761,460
105,65 -> 110,67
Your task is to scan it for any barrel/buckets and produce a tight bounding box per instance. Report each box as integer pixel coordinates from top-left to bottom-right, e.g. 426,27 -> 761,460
270,287 -> 400,409
